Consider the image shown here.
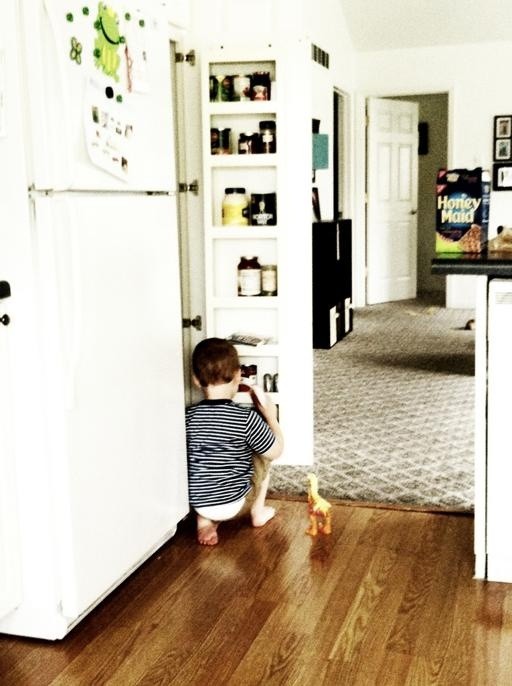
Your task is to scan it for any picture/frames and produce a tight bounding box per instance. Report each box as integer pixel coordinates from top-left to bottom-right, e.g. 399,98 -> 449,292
493,115 -> 512,191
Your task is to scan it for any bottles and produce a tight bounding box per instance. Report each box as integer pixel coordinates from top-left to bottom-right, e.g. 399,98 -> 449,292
235,254 -> 278,296
221,185 -> 251,226
210,70 -> 277,154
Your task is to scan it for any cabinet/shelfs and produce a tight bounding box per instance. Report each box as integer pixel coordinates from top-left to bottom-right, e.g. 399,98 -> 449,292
200,48 -> 277,405
313,219 -> 353,349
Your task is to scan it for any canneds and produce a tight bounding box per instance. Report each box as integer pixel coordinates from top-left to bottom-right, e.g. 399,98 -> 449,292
209,71 -> 277,296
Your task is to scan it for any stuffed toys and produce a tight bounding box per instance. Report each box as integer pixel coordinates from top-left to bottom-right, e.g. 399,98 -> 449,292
300,473 -> 332,536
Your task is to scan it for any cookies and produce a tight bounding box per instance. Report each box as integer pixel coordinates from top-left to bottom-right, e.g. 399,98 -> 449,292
457,226 -> 481,253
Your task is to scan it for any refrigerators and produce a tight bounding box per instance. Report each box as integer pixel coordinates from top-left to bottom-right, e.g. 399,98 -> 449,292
0,0 -> 319,646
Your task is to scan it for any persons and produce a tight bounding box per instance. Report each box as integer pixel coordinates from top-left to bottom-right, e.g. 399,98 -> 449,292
185,338 -> 284,545
